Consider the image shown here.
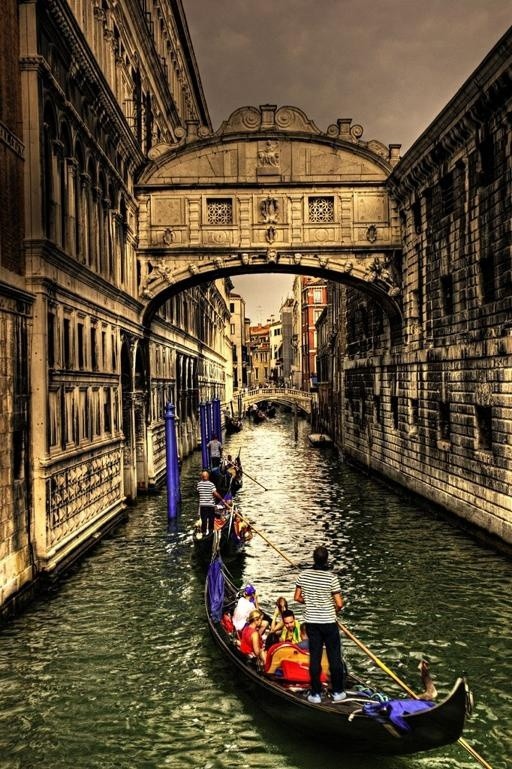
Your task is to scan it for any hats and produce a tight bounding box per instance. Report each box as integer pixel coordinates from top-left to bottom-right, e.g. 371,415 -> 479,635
244,584 -> 257,594
201,471 -> 209,479
246,610 -> 263,621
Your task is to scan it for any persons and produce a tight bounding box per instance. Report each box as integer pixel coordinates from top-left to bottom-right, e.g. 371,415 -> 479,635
221,455 -> 242,485
208,504 -> 252,544
212,255 -> 225,268
205,434 -> 222,470
231,583 -> 310,671
257,137 -> 281,166
294,252 -> 302,266
252,403 -> 258,414
343,260 -> 353,274
164,272 -> 177,284
319,254 -> 330,268
290,543 -> 345,707
267,247 -> 279,264
222,406 -> 230,422
189,264 -> 201,274
194,471 -> 224,538
240,253 -> 250,265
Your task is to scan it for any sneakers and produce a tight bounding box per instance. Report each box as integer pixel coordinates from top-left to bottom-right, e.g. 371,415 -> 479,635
331,691 -> 347,701
305,693 -> 322,704
